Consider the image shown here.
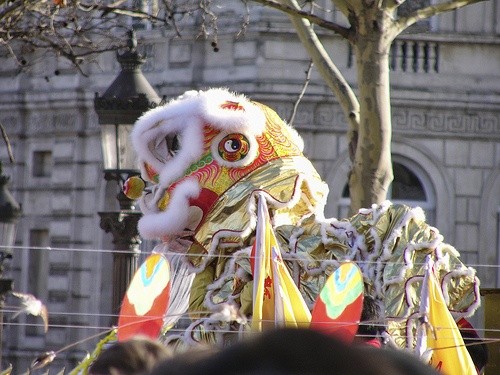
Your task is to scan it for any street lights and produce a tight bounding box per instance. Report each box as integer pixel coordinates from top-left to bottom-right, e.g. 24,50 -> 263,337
93,27 -> 168,328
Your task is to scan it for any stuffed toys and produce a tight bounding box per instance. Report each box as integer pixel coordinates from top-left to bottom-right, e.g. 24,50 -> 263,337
120,85 -> 482,366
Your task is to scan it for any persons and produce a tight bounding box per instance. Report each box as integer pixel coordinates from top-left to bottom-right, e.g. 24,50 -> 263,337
352,295 -> 382,350
